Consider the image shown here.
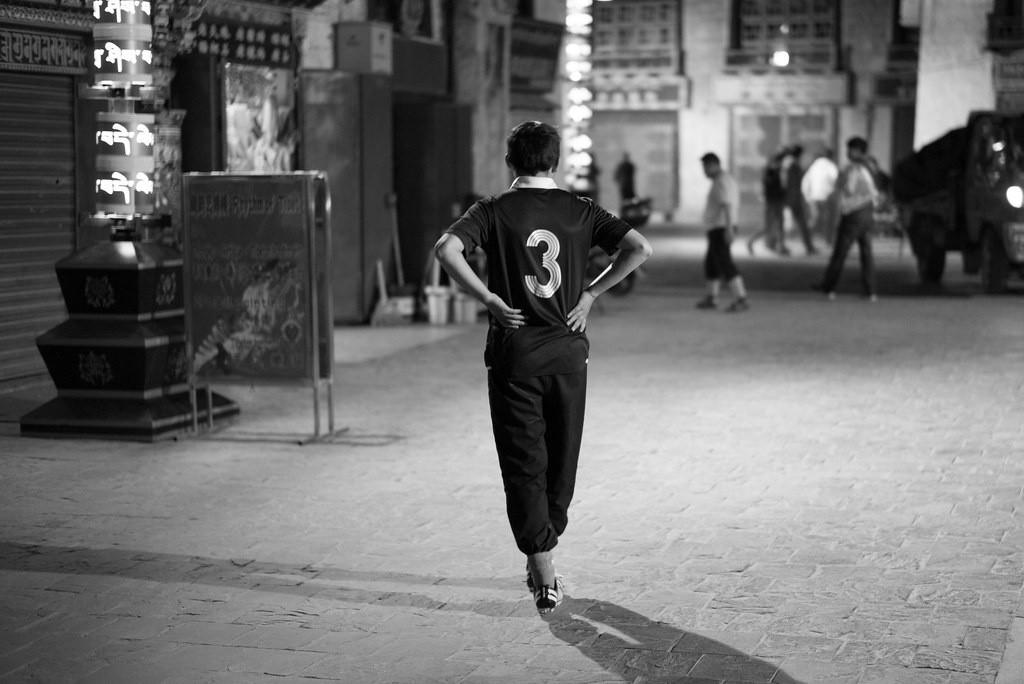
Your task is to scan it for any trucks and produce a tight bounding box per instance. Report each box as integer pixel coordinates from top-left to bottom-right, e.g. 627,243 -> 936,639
886,110 -> 1024,295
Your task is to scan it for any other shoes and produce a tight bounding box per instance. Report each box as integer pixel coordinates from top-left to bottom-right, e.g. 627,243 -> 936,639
860,289 -> 877,300
699,296 -> 717,307
729,300 -> 745,311
813,282 -> 832,294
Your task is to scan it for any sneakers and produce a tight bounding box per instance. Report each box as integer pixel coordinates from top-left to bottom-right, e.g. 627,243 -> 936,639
534,576 -> 565,614
522,571 -> 535,592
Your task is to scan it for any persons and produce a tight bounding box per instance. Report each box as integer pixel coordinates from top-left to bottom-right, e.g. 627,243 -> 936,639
819,138 -> 877,302
799,148 -> 842,245
695,154 -> 749,311
869,159 -> 892,194
612,154 -> 634,199
434,120 -> 653,611
749,145 -> 818,257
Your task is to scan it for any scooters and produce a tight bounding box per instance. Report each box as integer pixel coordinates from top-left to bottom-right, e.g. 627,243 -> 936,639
584,196 -> 654,299
